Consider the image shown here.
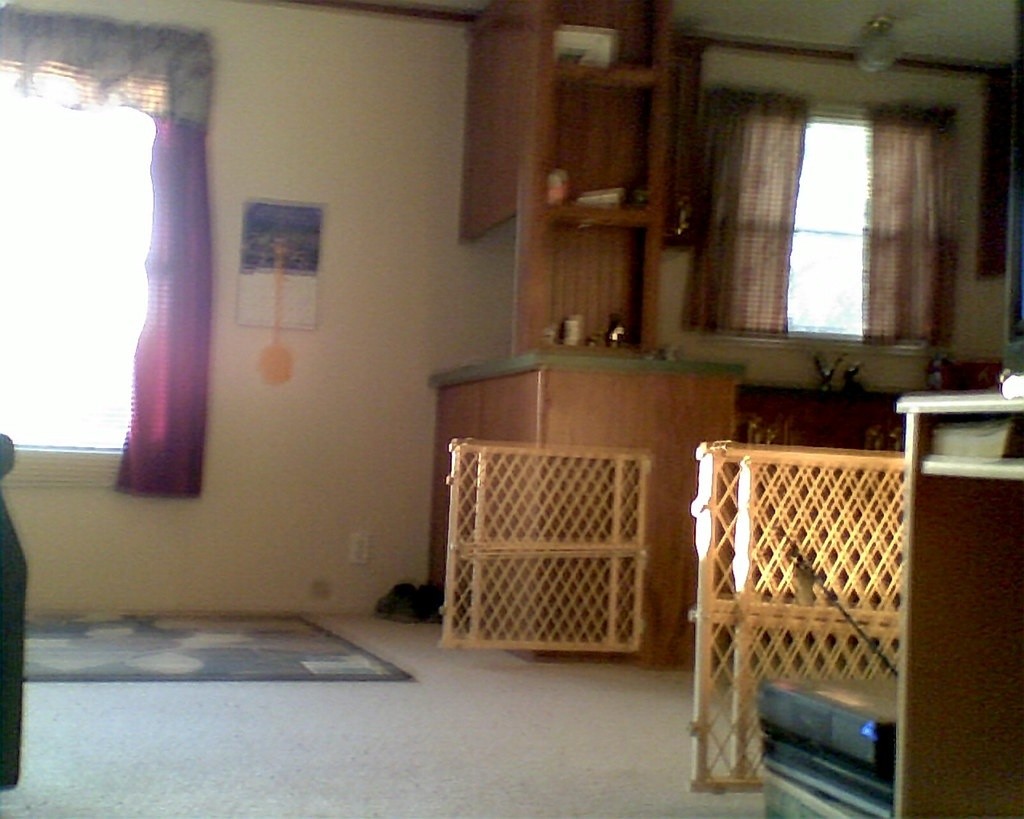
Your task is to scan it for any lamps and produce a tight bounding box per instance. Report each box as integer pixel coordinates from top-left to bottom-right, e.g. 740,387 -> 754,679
855,17 -> 896,74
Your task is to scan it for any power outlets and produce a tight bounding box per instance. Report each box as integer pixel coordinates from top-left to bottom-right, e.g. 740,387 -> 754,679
347,531 -> 369,565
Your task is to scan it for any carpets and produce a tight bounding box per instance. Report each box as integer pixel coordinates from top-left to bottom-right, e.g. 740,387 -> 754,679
25,607 -> 414,683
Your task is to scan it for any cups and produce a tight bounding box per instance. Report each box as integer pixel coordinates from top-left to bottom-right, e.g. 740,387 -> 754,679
546,169 -> 569,205
564,320 -> 580,347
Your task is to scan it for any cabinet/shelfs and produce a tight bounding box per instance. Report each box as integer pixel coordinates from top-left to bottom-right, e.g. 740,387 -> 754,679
737,383 -> 925,456
425,350 -> 740,664
887,388 -> 1024,818
460,0 -> 702,242
